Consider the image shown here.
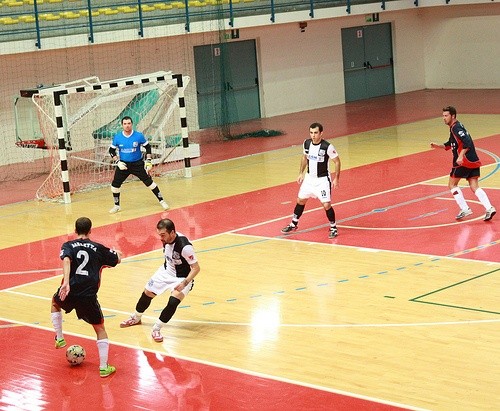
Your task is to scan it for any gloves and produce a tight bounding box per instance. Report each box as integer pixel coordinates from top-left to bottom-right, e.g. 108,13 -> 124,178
115,160 -> 127,170
144,158 -> 153,174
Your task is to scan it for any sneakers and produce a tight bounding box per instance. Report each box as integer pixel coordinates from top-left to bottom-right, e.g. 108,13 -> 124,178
483,207 -> 497,221
99,363 -> 116,377
159,201 -> 168,210
120,316 -> 142,328
109,204 -> 120,213
281,224 -> 297,234
456,207 -> 472,219
150,327 -> 163,341
55,335 -> 66,349
328,227 -> 338,239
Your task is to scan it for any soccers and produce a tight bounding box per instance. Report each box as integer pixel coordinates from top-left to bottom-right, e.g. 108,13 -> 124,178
65,344 -> 86,366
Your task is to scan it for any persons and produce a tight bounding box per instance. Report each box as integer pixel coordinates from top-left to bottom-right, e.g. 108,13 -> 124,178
109,117 -> 169,215
119,218 -> 200,342
431,106 -> 497,221
280,123 -> 340,240
51,218 -> 122,378
